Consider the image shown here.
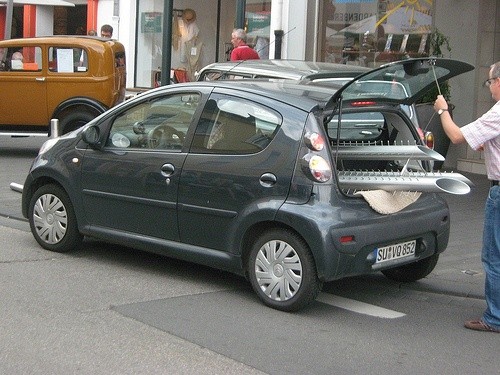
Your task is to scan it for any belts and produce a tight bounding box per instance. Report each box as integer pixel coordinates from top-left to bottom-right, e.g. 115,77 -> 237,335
490,180 -> 500,186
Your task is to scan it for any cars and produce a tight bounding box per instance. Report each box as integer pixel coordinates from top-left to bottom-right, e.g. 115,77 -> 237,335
0,34 -> 126,140
22,57 -> 476,313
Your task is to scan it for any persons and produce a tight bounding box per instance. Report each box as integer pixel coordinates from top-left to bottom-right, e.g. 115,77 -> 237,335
327,38 -> 388,68
432,63 -> 500,332
12,52 -> 23,62
79,25 -> 113,66
230,29 -> 260,60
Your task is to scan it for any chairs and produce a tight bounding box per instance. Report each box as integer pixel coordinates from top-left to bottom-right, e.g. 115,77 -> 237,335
222,106 -> 259,141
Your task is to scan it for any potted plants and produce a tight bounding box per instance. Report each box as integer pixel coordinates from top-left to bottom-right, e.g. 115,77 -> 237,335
413,30 -> 456,171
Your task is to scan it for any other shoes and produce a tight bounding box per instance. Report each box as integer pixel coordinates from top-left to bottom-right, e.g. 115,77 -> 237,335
464,319 -> 500,333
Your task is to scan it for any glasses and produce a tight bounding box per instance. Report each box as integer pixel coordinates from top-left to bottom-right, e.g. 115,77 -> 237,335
485,76 -> 497,85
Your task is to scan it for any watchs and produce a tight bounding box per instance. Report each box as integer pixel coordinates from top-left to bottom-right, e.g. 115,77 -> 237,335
438,109 -> 448,116
